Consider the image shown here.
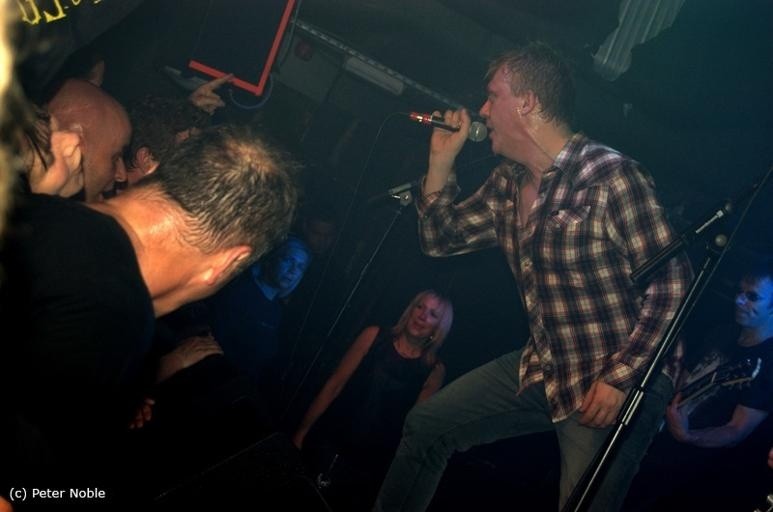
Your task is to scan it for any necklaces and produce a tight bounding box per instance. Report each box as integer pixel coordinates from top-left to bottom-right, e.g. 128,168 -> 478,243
397,339 -> 418,358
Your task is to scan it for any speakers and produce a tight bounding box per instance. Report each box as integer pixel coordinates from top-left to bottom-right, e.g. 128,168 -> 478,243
188,0 -> 297,96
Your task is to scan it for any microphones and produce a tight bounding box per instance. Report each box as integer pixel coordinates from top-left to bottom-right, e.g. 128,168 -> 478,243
408,110 -> 488,142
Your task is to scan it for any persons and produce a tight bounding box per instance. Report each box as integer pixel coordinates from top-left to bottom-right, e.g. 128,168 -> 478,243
650,260 -> 773,511
285,287 -> 455,511
0,39 -> 338,512
376,44 -> 693,512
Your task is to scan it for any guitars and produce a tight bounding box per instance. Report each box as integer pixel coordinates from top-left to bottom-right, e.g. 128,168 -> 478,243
668,350 -> 762,410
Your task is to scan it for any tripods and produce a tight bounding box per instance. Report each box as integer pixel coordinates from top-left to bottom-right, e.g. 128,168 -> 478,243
152,193 -> 413,511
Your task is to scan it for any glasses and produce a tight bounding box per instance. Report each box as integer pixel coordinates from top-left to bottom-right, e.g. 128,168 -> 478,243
735,287 -> 767,302
